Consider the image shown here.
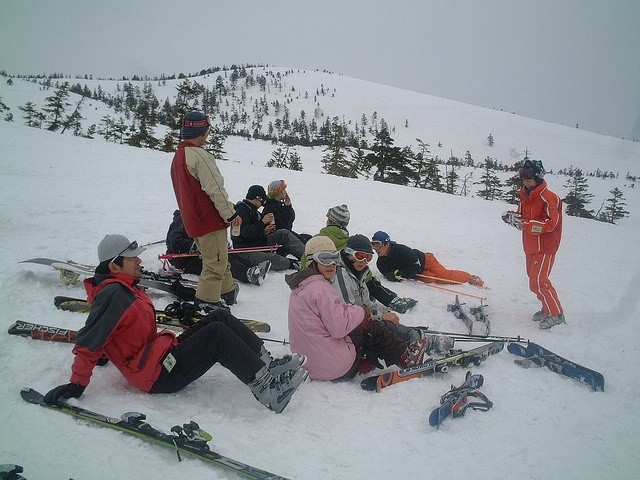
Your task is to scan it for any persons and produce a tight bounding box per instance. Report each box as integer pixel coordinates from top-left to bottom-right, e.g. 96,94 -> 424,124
330,233 -> 458,354
230,184 -> 305,270
263,180 -> 311,241
298,203 -> 417,314
43,234 -> 309,414
167,211 -> 271,287
285,236 -> 428,382
371,231 -> 486,289
502,158 -> 566,330
170,112 -> 243,314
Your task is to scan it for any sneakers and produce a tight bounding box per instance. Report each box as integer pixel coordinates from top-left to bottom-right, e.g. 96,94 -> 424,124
389,297 -> 417,313
220,284 -> 240,306
469,275 -> 485,286
249,367 -> 308,412
400,339 -> 426,367
289,258 -> 301,270
260,344 -> 307,376
246,260 -> 271,286
539,314 -> 566,330
195,299 -> 228,315
426,335 -> 454,353
532,311 -> 550,321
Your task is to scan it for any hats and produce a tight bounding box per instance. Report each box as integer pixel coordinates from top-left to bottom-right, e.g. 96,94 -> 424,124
328,204 -> 350,227
248,185 -> 269,201
98,235 -> 146,262
346,235 -> 375,254
181,112 -> 212,140
305,236 -> 341,269
269,180 -> 287,197
372,231 -> 390,243
519,160 -> 546,183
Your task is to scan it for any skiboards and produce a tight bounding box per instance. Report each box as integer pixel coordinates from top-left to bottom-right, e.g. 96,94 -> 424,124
17,257 -> 198,301
360,342 -> 504,393
20,387 -> 290,480
507,342 -> 604,392
429,371 -> 484,430
54,296 -> 270,333
447,294 -> 490,336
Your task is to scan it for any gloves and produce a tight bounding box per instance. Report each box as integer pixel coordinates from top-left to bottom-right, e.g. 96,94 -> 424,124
362,304 -> 372,320
501,210 -> 524,231
43,383 -> 87,405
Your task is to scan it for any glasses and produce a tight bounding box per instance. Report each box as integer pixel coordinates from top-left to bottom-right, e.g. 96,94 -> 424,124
371,241 -> 382,249
110,240 -> 138,262
344,246 -> 373,264
307,250 -> 341,266
